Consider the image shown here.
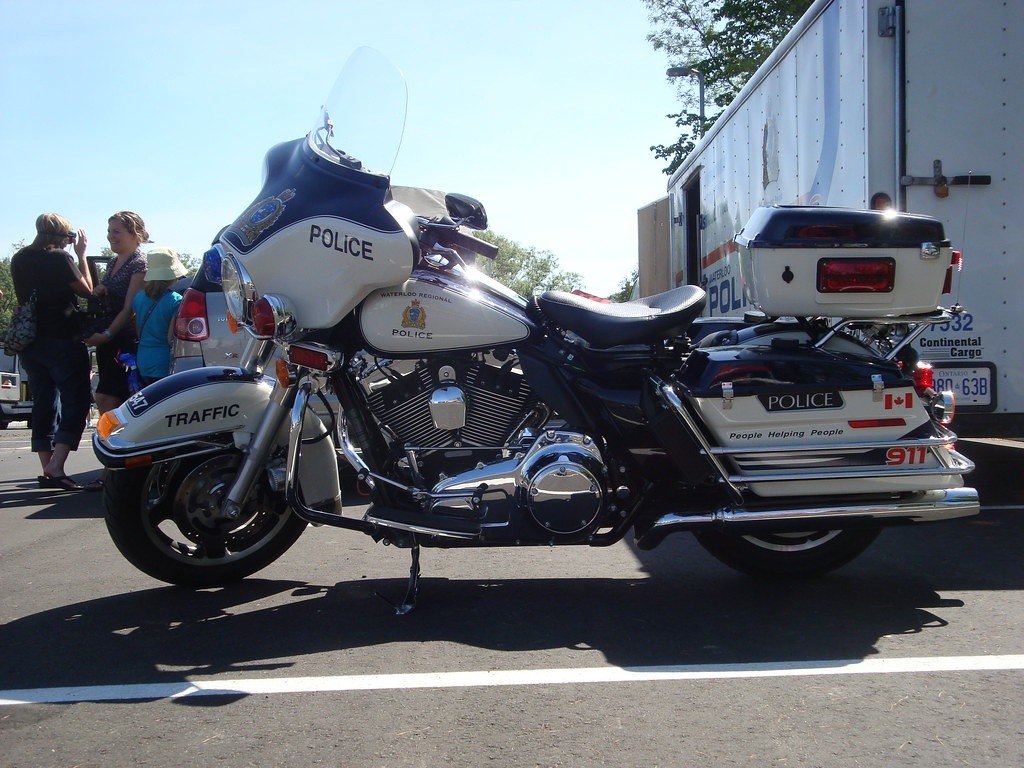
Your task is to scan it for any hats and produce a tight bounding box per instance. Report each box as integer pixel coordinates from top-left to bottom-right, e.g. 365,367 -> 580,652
144,247 -> 189,281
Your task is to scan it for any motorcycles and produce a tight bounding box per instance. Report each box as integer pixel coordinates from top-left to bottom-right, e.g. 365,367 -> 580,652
92,45 -> 981,604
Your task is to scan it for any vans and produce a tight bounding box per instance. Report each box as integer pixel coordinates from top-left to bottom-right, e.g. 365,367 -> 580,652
0,343 -> 36,434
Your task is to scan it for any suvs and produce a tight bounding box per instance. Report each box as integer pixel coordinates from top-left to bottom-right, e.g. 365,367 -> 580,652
86,224 -> 359,450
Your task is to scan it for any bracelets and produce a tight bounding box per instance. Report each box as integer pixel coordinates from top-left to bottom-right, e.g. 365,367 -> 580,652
102,330 -> 112,340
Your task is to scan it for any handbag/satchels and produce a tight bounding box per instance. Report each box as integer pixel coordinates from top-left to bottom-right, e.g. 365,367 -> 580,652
81,284 -> 113,341
118,353 -> 145,395
3,301 -> 37,357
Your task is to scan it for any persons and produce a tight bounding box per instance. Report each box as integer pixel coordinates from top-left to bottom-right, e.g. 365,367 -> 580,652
4,210 -> 190,491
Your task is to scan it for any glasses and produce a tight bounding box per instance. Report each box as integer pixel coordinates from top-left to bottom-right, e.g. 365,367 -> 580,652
39,230 -> 76,244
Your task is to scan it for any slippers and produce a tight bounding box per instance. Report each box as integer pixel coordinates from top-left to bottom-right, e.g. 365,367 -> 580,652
37,474 -> 84,491
85,478 -> 105,492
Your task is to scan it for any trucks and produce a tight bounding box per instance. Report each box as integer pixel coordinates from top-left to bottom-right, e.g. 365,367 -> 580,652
668,0 -> 1023,508
